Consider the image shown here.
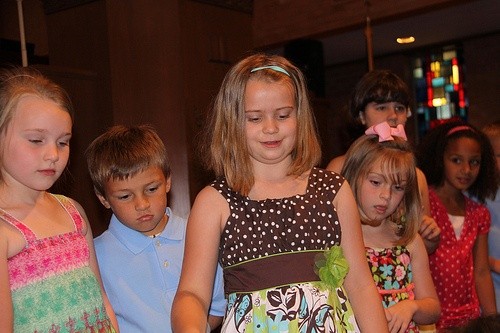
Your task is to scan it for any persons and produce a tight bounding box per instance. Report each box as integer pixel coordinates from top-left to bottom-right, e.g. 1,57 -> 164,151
428,120 -> 500,333
171,55 -> 389,333
339,133 -> 440,333
326,68 -> 441,255
84,125 -> 227,333
0,66 -> 119,333
